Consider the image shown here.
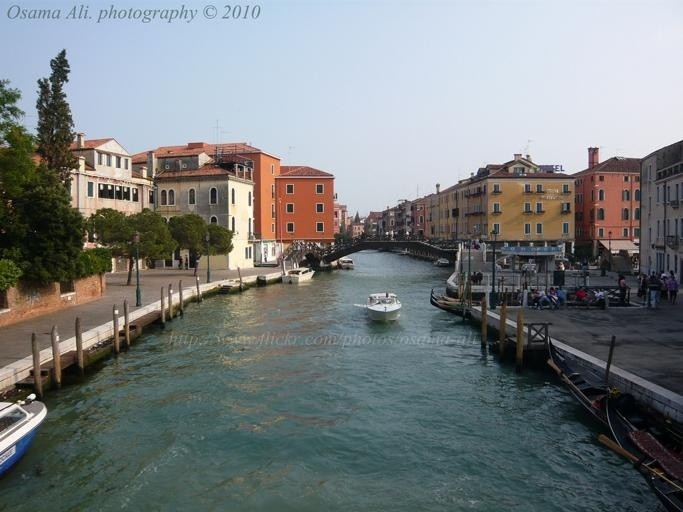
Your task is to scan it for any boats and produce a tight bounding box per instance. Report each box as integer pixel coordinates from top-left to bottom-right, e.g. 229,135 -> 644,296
338,257 -> 354,269
0,393 -> 48,474
434,259 -> 449,267
546,333 -> 683,512
366,291 -> 402,323
282,267 -> 315,284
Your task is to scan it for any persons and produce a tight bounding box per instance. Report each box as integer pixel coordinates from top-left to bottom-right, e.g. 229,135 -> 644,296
476,271 -> 483,286
470,271 -> 478,286
382,291 -> 392,303
185,254 -> 189,270
513,283 -> 610,312
619,276 -> 630,303
559,262 -> 565,271
636,268 -> 679,307
178,255 -> 184,270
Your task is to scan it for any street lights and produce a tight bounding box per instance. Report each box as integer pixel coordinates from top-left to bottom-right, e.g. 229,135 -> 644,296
205,230 -> 212,283
132,229 -> 143,306
488,229 -> 498,310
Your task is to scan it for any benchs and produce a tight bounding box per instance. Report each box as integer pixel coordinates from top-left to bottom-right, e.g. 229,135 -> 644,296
522,289 -> 607,310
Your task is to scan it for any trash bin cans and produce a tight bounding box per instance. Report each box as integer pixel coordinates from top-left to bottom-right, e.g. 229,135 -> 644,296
646,284 -> 661,306
